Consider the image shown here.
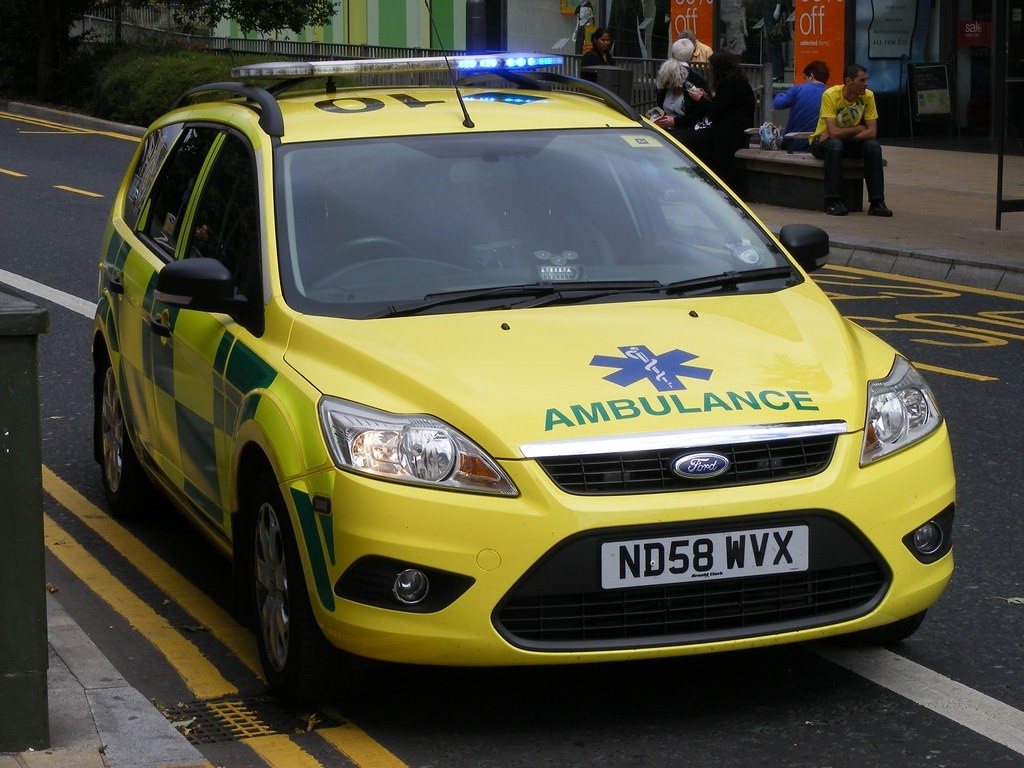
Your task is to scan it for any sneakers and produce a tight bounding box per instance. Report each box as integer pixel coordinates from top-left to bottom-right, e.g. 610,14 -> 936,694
824,198 -> 848,215
868,201 -> 892,217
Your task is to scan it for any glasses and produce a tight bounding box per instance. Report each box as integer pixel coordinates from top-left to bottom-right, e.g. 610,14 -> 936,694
803,74 -> 807,79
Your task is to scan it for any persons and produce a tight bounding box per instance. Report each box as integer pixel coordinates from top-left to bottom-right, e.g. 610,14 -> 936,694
580,27 -> 617,82
657,31 -> 755,173
193,223 -> 211,257
720,0 -> 749,73
761,0 -> 791,83
773,60 -> 829,150
571,0 -> 595,54
809,63 -> 893,217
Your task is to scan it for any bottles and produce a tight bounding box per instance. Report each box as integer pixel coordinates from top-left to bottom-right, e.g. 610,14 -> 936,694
684,81 -> 698,92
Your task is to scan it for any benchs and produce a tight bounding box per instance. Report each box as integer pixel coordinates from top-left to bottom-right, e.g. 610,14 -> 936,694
734,127 -> 887,212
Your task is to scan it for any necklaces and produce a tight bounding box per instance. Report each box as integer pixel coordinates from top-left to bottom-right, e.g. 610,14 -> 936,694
842,86 -> 859,109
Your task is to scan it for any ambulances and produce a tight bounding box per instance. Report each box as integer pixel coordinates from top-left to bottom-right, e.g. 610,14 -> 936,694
89,50 -> 958,715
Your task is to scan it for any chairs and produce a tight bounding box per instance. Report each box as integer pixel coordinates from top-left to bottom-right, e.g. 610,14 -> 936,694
452,159 -> 616,269
237,165 -> 364,297
